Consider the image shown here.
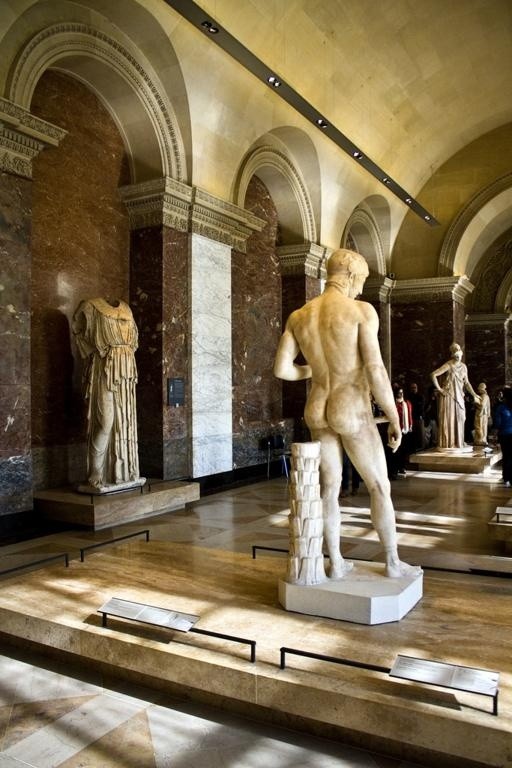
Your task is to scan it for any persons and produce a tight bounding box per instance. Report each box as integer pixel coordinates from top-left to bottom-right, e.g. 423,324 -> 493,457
270,248 -> 426,581
475,381 -> 492,445
359,378 -> 438,480
71,296 -> 147,494
495,383 -> 512,489
429,342 -> 483,448
